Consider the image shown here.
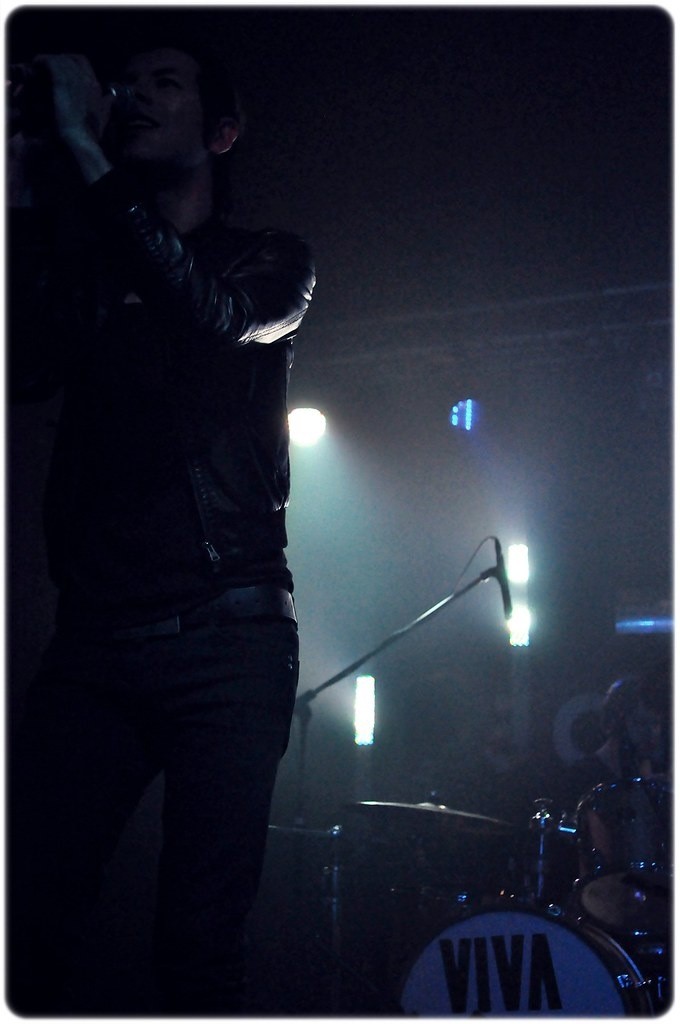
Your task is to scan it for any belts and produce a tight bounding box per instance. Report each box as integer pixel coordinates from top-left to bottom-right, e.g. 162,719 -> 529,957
58,586 -> 296,644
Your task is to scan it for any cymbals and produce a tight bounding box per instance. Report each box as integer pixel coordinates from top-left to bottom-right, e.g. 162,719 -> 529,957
357,803 -> 511,828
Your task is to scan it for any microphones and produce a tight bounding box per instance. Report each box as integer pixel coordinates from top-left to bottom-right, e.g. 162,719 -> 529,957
495,538 -> 512,620
8,63 -> 136,116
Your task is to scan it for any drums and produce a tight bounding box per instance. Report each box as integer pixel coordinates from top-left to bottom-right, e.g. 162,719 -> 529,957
573,774 -> 672,926
402,905 -> 650,1017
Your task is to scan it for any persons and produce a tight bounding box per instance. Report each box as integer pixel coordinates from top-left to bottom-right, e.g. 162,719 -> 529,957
4,33 -> 317,1017
575,660 -> 670,881
